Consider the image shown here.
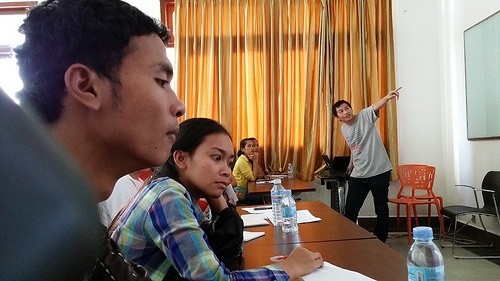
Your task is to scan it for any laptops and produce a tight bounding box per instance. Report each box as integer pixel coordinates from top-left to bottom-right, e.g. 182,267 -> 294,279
320,154 -> 351,178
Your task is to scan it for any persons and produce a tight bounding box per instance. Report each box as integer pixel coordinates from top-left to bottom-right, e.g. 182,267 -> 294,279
0,0 -> 186,281
108,117 -> 324,281
98,137 -> 267,266
331,86 -> 403,243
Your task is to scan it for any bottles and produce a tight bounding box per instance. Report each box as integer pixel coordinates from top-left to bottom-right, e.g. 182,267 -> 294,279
287,163 -> 293,180
281,190 -> 300,258
271,180 -> 285,227
407,226 -> 445,281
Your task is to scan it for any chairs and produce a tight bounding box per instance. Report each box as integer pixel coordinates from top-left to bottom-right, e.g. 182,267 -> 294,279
388,164 -> 446,245
440,171 -> 500,259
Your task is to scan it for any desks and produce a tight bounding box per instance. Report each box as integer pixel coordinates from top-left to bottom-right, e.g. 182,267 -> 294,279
236,200 -> 377,248
248,176 -> 316,204
236,238 -> 407,281
